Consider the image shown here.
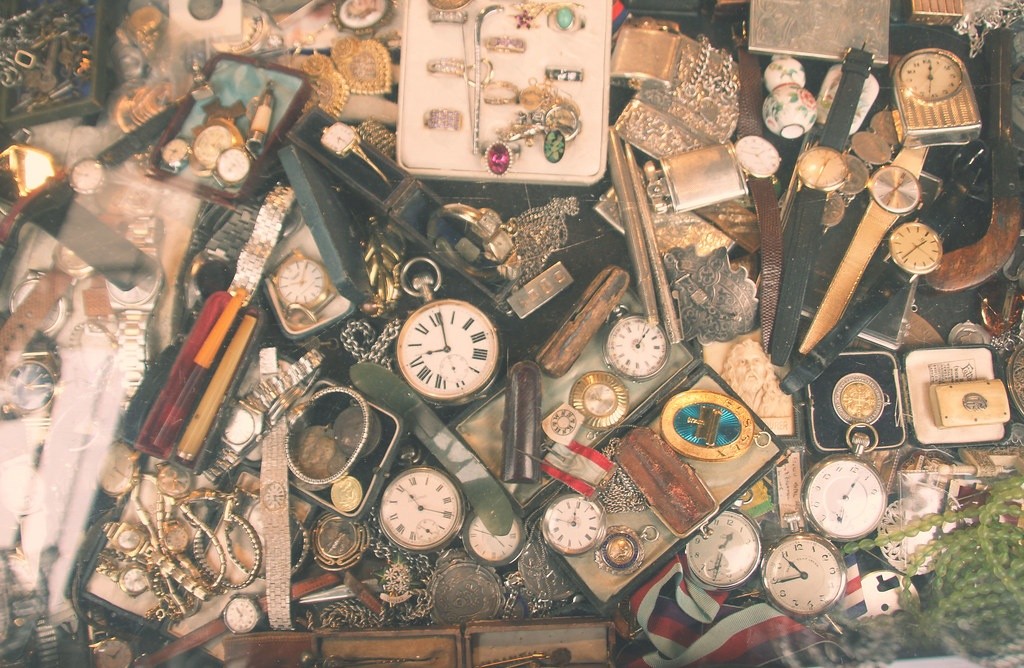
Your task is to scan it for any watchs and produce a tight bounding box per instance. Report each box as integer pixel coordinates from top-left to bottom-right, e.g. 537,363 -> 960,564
0,0 -> 1024,667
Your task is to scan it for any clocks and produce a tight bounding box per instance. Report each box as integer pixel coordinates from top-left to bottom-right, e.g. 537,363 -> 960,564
374,463 -> 465,556
537,493 -> 603,556
392,258 -> 507,409
893,48 -> 985,148
602,315 -> 670,380
683,421 -> 888,621
463,501 -> 529,570
